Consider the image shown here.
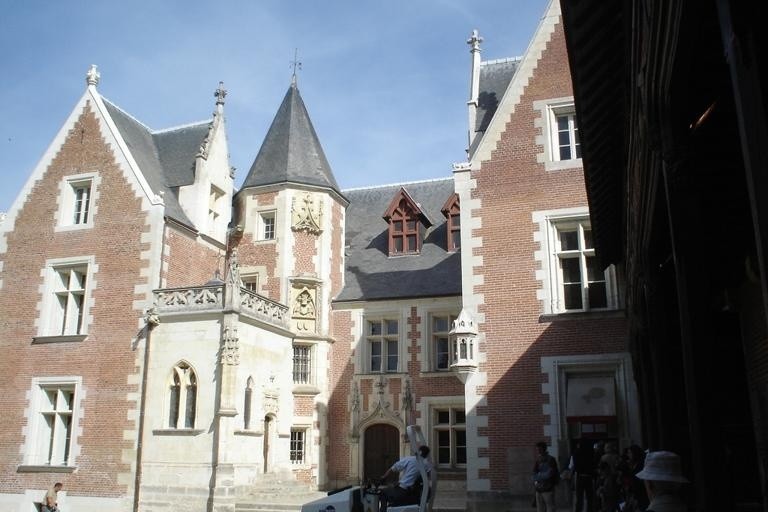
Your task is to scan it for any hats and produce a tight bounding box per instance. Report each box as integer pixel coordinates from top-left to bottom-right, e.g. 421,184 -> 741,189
635,449 -> 694,487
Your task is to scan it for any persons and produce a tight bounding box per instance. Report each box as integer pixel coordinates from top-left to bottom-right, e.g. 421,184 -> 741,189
531,441 -> 560,512
567,437 -> 694,512
39,482 -> 62,512
377,444 -> 435,512
